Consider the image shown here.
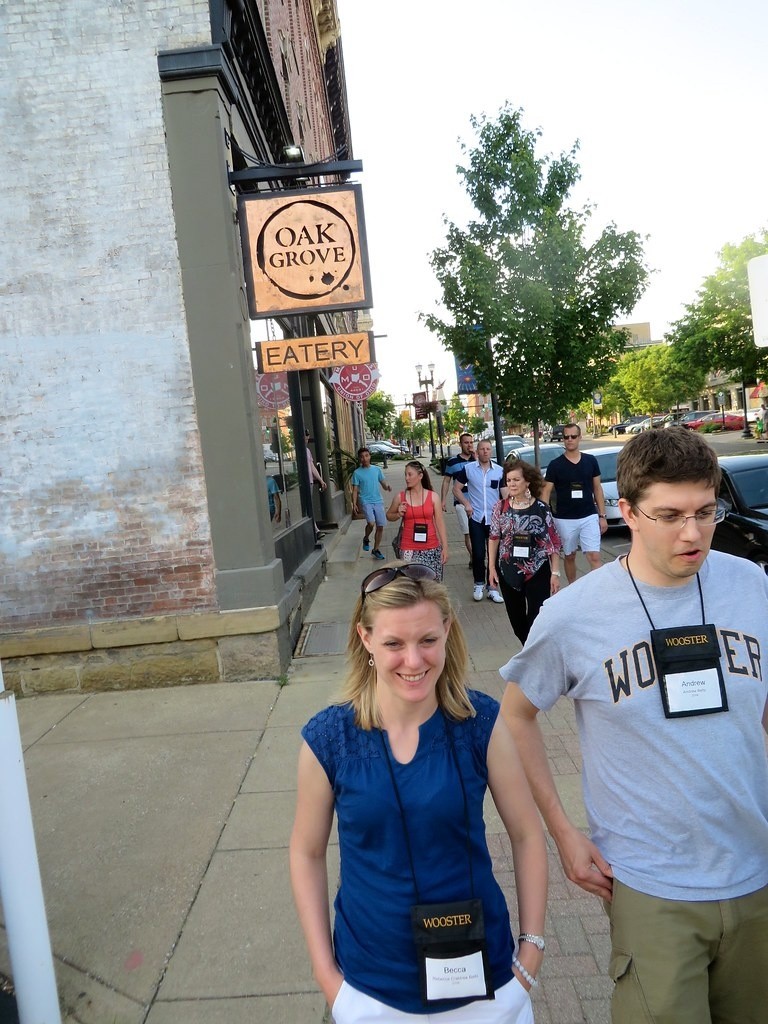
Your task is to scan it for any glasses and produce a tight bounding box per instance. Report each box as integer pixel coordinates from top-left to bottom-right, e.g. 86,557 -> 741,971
360,563 -> 438,603
563,435 -> 579,439
633,498 -> 728,531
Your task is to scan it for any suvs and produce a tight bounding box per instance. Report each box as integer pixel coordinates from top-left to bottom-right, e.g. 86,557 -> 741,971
608,414 -> 650,435
365,440 -> 409,458
550,424 -> 567,442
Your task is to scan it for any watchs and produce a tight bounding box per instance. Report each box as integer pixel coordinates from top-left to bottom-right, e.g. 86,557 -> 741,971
552,571 -> 561,577
517,934 -> 546,952
599,514 -> 607,519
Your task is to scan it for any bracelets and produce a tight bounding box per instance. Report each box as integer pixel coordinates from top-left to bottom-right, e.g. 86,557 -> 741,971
512,956 -> 539,987
397,513 -> 401,518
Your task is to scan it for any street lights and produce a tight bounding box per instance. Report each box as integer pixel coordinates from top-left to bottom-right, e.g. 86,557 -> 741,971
415,362 -> 438,466
739,329 -> 755,439
403,393 -> 415,458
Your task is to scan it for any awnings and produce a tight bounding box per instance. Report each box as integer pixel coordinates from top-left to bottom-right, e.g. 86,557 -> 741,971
749,380 -> 768,398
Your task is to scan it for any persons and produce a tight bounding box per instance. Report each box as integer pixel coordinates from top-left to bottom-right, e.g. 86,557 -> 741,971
387,438 -> 426,453
351,448 -> 392,559
754,404 -> 768,439
289,561 -> 548,1024
441,434 -> 486,570
488,459 -> 565,647
452,439 -> 510,603
540,423 -> 609,586
305,427 -> 327,540
386,461 -> 449,583
264,461 -> 282,523
498,425 -> 768,1024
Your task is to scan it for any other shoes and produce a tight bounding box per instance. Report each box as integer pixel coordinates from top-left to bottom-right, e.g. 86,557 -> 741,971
473,583 -> 485,601
487,590 -> 504,603
362,536 -> 370,551
316,531 -> 326,539
370,548 -> 385,560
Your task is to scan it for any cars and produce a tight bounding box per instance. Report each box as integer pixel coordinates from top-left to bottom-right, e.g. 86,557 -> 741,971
717,454 -> 768,572
625,416 -> 664,434
505,443 -> 566,477
664,410 -> 720,429
581,444 -> 630,529
725,407 -> 768,422
682,412 -> 745,432
490,434 -> 529,461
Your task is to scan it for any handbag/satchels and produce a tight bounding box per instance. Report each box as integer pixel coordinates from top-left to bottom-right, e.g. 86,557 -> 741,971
392,526 -> 401,559
